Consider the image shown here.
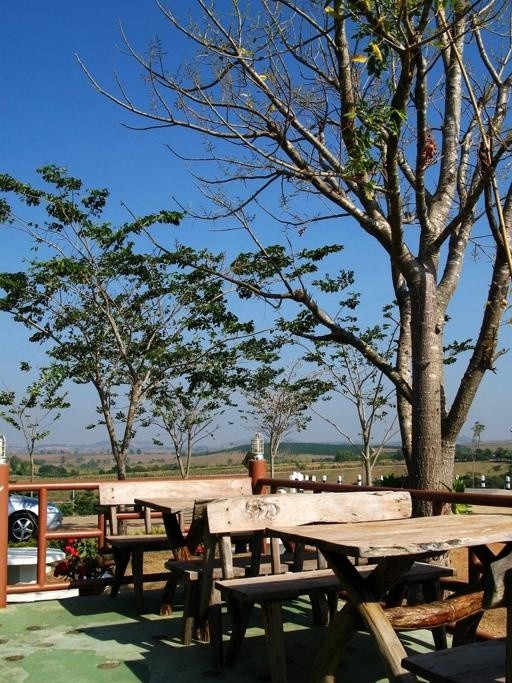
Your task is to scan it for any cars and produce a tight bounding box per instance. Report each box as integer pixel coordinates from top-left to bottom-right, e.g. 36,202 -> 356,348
8,493 -> 62,543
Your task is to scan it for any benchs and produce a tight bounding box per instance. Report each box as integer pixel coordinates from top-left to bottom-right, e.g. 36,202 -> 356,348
100,477 -> 512,683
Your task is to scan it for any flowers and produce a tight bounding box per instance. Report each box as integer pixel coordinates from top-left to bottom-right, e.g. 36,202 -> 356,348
53,539 -> 114,586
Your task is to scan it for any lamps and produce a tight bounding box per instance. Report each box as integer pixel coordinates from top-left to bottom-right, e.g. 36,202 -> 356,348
251,433 -> 265,460
0,433 -> 7,464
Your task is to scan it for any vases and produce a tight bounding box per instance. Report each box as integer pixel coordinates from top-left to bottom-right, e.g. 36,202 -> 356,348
76,576 -> 105,596
